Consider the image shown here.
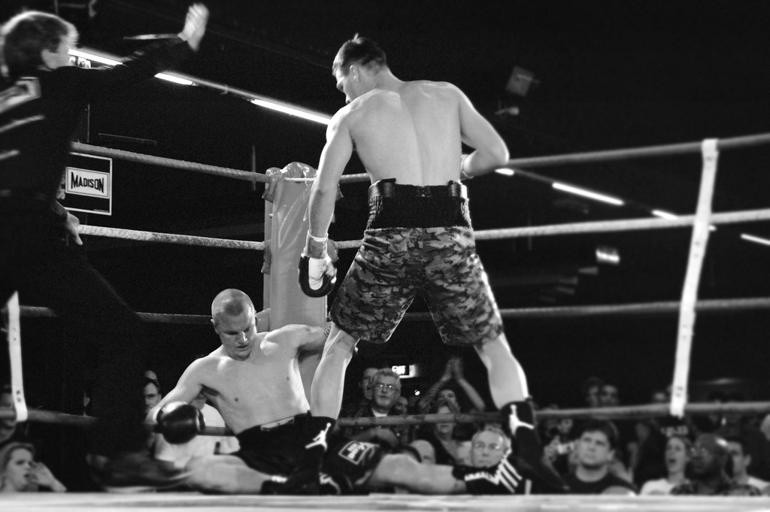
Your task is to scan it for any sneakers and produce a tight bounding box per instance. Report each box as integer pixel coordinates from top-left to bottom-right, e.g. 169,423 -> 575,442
98,456 -> 198,495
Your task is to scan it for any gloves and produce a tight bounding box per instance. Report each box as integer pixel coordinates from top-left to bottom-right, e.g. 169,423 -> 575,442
296,228 -> 338,299
155,400 -> 207,446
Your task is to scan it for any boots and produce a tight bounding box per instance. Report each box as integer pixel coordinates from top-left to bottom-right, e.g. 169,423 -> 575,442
497,394 -> 576,494
452,445 -> 534,495
257,467 -> 343,498
259,415 -> 338,497
